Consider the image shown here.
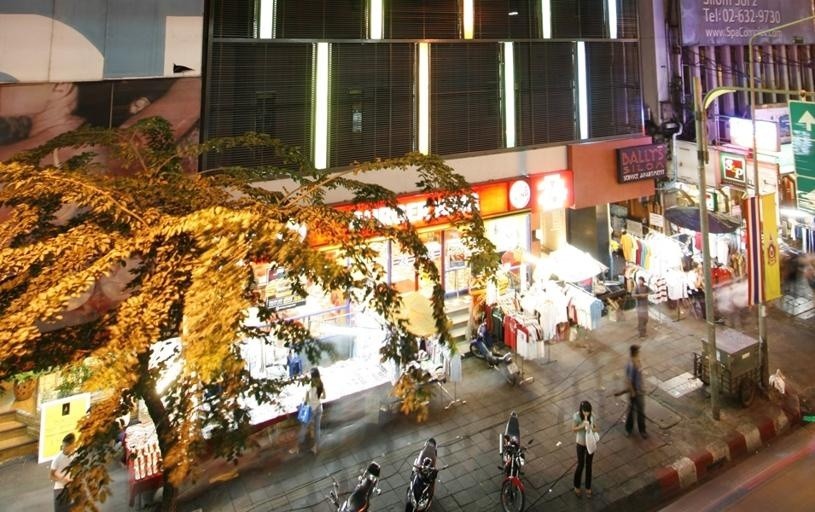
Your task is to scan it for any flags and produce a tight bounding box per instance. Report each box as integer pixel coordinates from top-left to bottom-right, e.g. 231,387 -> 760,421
760,192 -> 783,302
740,195 -> 767,306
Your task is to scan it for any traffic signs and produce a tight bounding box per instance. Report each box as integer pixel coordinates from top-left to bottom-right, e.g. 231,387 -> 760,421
788,101 -> 815,214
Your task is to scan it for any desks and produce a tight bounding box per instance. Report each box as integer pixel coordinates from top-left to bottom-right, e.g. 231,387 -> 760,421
125,357 -> 394,511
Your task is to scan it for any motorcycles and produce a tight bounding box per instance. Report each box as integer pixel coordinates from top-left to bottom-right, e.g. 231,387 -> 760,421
468,320 -> 521,388
404,436 -> 439,512
324,460 -> 384,512
496,409 -> 534,512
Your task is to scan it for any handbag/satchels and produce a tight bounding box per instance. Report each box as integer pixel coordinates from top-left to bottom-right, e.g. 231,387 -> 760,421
585,431 -> 598,455
297,404 -> 312,425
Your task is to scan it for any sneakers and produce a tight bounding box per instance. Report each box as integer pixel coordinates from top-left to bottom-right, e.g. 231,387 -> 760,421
575,487 -> 582,497
310,446 -> 318,454
585,487 -> 592,498
624,428 -> 632,437
639,431 -> 648,439
289,447 -> 300,455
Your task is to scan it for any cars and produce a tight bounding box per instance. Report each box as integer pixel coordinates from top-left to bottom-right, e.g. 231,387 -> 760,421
735,225 -> 805,279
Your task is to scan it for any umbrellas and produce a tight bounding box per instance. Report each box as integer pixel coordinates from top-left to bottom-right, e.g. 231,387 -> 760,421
663,204 -> 741,238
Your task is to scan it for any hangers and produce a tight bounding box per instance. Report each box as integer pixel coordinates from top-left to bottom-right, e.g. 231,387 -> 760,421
617,222 -> 679,258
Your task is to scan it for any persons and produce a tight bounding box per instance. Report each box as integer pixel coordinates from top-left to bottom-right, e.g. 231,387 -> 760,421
50,433 -> 79,511
1,77 -> 203,237
623,344 -> 650,439
287,366 -> 327,456
475,318 -> 504,357
571,399 -> 600,499
686,263 -> 724,324
631,276 -> 656,341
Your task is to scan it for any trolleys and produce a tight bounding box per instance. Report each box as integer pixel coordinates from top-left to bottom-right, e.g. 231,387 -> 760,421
692,327 -> 764,407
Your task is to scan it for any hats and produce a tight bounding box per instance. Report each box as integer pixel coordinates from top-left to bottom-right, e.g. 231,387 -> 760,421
638,276 -> 646,283
